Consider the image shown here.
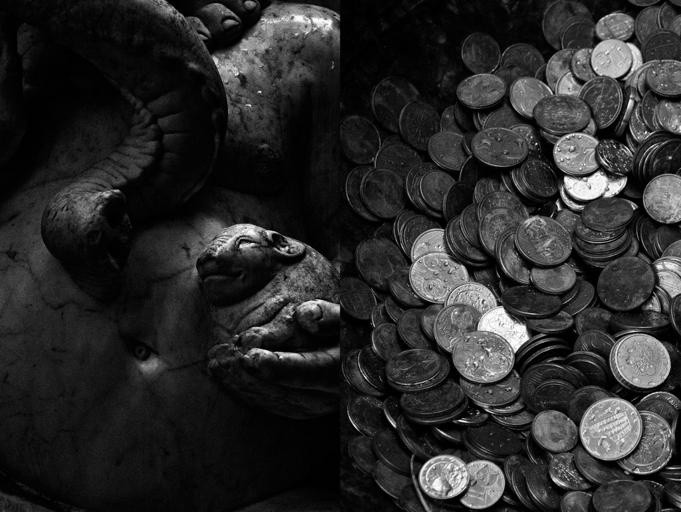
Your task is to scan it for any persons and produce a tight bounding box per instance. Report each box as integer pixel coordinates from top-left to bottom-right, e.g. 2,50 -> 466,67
200,294 -> 344,426
183,0 -> 261,49
